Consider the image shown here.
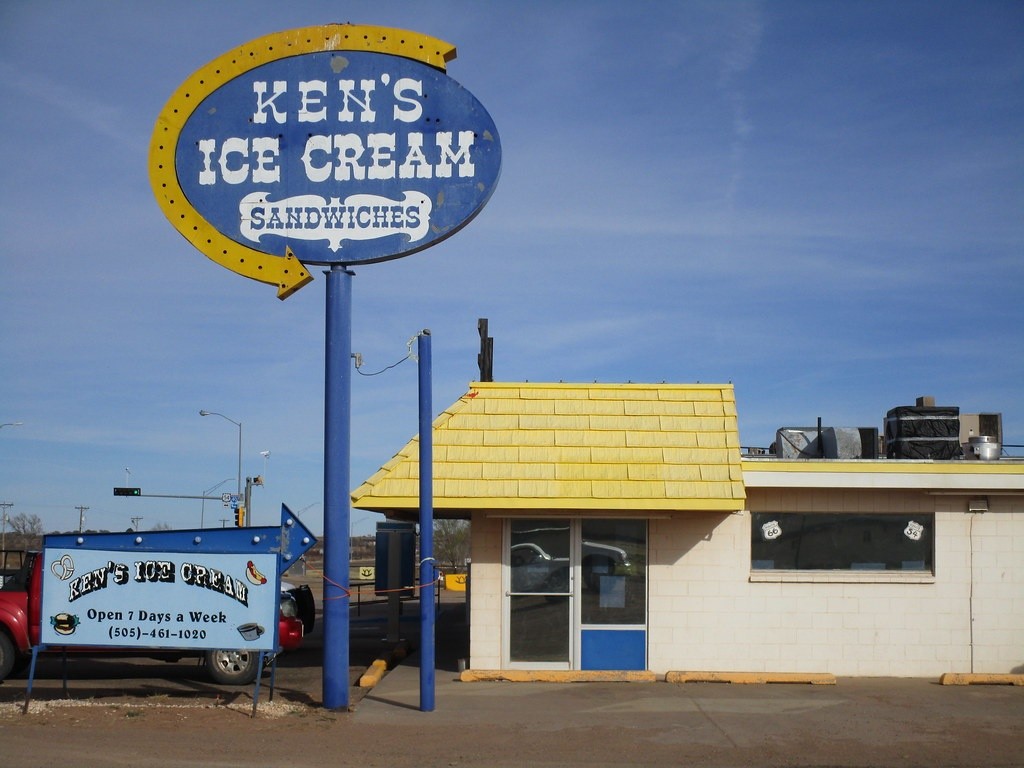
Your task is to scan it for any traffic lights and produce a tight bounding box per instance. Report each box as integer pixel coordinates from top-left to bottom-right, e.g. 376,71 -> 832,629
113,488 -> 141,497
253,476 -> 262,483
234,506 -> 244,527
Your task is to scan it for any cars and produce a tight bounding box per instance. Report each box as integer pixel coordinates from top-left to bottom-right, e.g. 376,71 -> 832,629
510,542 -> 571,604
581,540 -> 631,592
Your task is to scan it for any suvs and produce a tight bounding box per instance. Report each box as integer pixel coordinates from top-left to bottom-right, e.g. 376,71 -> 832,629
0,551 -> 305,685
280,579 -> 314,635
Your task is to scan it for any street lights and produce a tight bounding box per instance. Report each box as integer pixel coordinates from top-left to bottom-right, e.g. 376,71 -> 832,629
201,478 -> 238,530
198,409 -> 242,502
